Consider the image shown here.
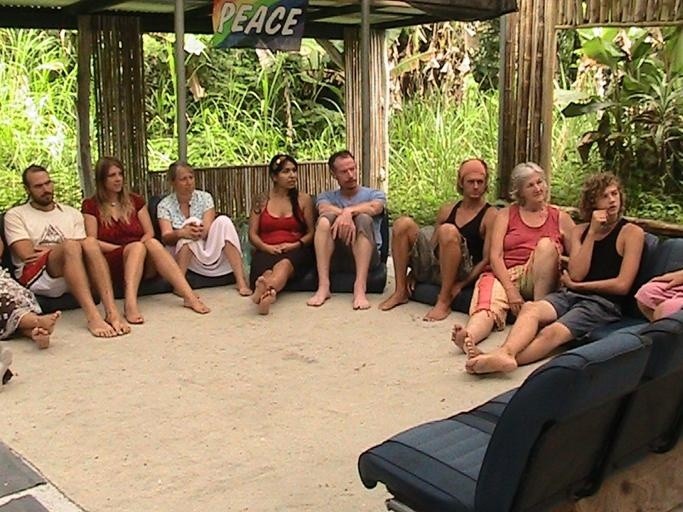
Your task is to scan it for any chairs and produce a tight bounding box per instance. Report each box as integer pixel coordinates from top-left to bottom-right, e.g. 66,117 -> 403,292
241,208 -> 390,293
357,229 -> 683,512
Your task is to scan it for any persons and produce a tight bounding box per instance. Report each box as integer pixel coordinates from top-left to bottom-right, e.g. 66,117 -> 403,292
248,155 -> 317,316
0,237 -> 62,349
82,157 -> 211,324
307,150 -> 386,310
463,173 -> 645,374
3,165 -> 131,337
157,161 -> 252,296
452,161 -> 577,358
634,269 -> 683,323
379,158 -> 498,321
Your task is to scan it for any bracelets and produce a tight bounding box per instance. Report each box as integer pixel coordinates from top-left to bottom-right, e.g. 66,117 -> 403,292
297,239 -> 304,248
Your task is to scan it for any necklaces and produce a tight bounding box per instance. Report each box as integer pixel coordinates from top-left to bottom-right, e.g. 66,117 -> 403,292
111,203 -> 117,206
